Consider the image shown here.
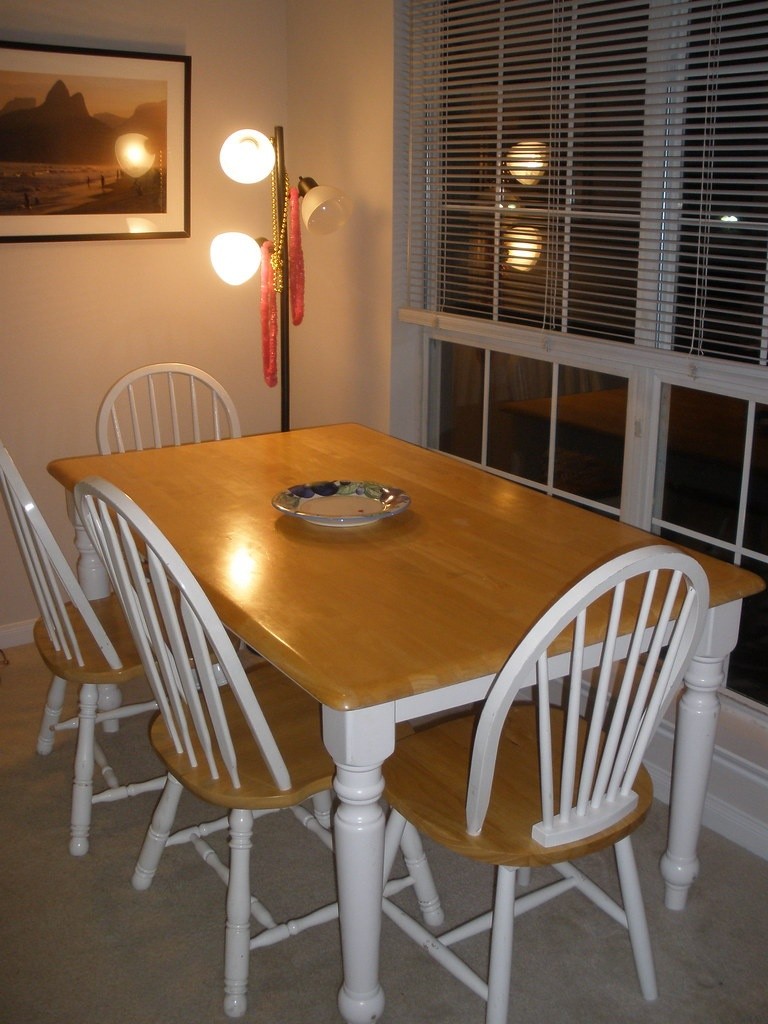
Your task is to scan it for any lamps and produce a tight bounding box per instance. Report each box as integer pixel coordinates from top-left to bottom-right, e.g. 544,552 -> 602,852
114,133 -> 166,178
207,125 -> 352,431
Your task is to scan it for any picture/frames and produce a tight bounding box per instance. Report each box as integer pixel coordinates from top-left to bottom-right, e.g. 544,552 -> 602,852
0,36 -> 191,244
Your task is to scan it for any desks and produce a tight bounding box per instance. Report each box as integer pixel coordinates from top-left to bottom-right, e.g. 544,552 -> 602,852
52,426 -> 763,1023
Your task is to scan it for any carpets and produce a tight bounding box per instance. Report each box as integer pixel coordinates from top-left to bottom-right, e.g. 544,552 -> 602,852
2,683 -> 766,1024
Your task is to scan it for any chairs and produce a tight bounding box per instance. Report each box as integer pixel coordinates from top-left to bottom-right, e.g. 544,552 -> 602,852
72,479 -> 443,1017
381,542 -> 708,1024
96,363 -> 242,457
0,446 -> 239,856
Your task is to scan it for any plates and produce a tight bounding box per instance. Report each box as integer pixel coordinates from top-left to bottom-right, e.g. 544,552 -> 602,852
270,479 -> 411,527
